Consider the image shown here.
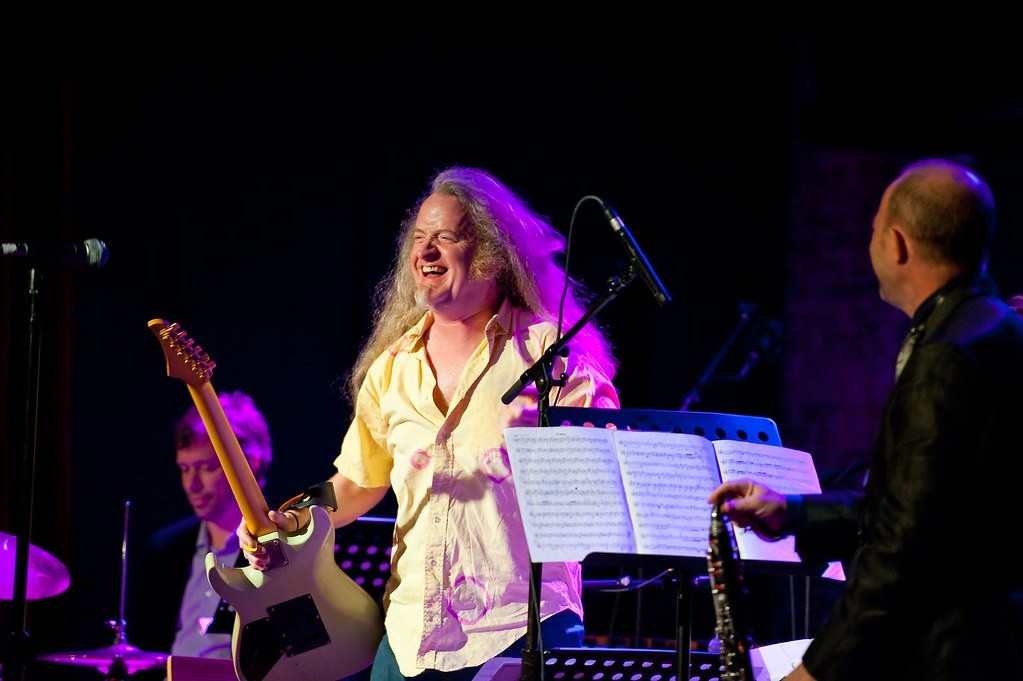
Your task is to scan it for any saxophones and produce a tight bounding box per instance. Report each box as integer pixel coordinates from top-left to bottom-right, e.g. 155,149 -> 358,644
705,498 -> 757,681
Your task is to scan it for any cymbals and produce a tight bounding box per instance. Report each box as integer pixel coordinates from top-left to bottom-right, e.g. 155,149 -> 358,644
37,643 -> 172,677
0,531 -> 72,601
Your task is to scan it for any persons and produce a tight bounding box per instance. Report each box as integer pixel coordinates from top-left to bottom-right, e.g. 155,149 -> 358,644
125,390 -> 272,680
235,167 -> 622,681
706,158 -> 1023,681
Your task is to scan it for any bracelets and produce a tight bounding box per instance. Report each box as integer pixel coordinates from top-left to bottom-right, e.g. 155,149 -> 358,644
284,511 -> 300,532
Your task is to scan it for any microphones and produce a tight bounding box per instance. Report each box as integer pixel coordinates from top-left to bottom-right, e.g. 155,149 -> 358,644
598,202 -> 672,308
0,239 -> 108,267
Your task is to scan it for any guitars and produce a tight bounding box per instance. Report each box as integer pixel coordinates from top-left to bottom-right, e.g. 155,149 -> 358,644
147,318 -> 385,681
